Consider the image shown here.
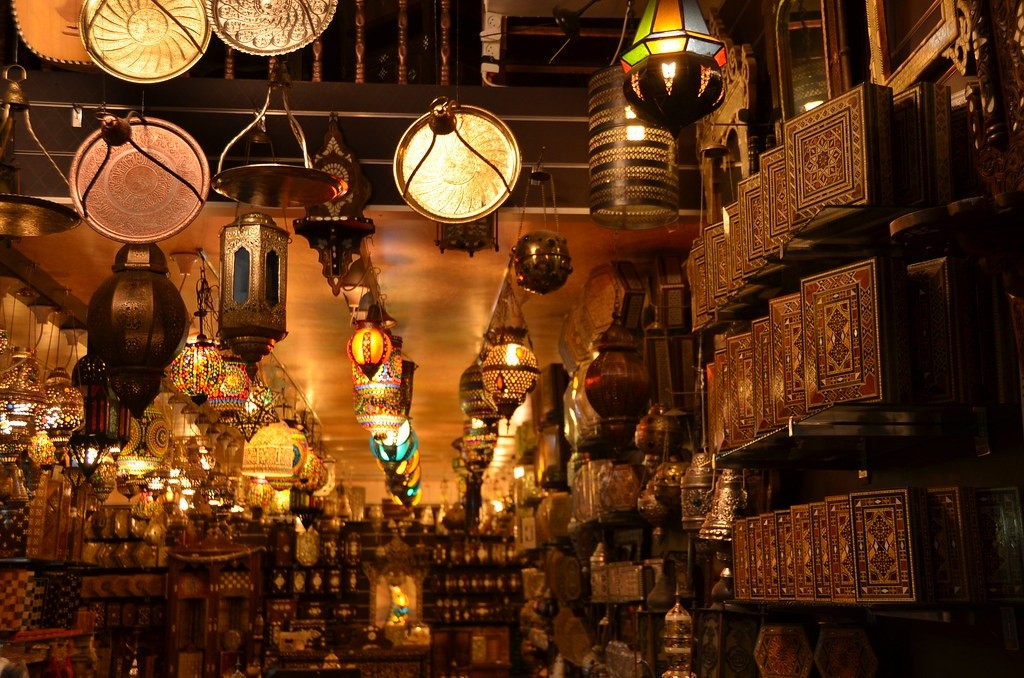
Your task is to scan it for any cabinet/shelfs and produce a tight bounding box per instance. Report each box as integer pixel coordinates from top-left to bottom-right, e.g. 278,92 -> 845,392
691,203 -> 1024,621
240,503 -> 530,678
82,565 -> 175,678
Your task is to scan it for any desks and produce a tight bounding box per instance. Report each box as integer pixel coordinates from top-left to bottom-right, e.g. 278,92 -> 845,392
276,622 -> 433,678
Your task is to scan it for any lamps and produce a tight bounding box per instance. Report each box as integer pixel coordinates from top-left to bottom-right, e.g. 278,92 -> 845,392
0,0 -> 731,678
